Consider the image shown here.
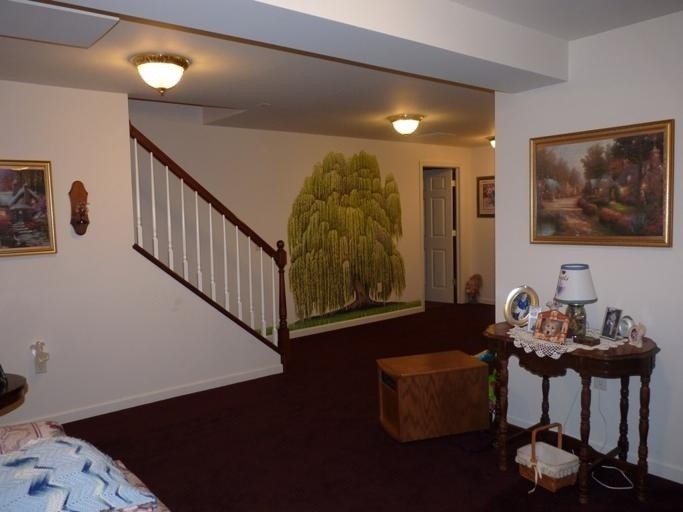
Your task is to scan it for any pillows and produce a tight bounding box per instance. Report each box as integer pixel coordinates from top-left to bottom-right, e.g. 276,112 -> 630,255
1,434 -> 159,511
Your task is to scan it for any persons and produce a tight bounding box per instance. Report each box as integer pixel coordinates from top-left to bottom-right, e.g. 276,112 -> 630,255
515,293 -> 529,320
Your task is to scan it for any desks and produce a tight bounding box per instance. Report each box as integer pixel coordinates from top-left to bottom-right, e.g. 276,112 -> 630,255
0,371 -> 28,417
477,317 -> 666,511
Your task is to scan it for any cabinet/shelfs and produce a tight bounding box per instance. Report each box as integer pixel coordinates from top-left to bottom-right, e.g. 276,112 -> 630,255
373,349 -> 493,443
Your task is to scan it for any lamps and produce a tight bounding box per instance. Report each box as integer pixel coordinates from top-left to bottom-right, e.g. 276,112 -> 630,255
382,112 -> 428,138
125,49 -> 194,101
550,260 -> 599,340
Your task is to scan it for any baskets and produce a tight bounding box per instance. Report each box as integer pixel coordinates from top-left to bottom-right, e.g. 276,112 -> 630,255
515,423 -> 581,491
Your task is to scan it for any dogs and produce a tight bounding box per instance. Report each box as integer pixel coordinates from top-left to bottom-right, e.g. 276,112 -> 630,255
541,319 -> 562,337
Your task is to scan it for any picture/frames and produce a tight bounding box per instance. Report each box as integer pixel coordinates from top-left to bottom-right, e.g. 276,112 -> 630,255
625,322 -> 647,349
531,308 -> 571,345
597,305 -> 624,341
617,316 -> 636,338
527,116 -> 677,249
504,282 -> 540,329
475,174 -> 495,219
0,159 -> 57,259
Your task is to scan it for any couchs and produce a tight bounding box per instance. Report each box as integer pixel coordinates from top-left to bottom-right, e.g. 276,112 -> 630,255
0,416 -> 174,512
